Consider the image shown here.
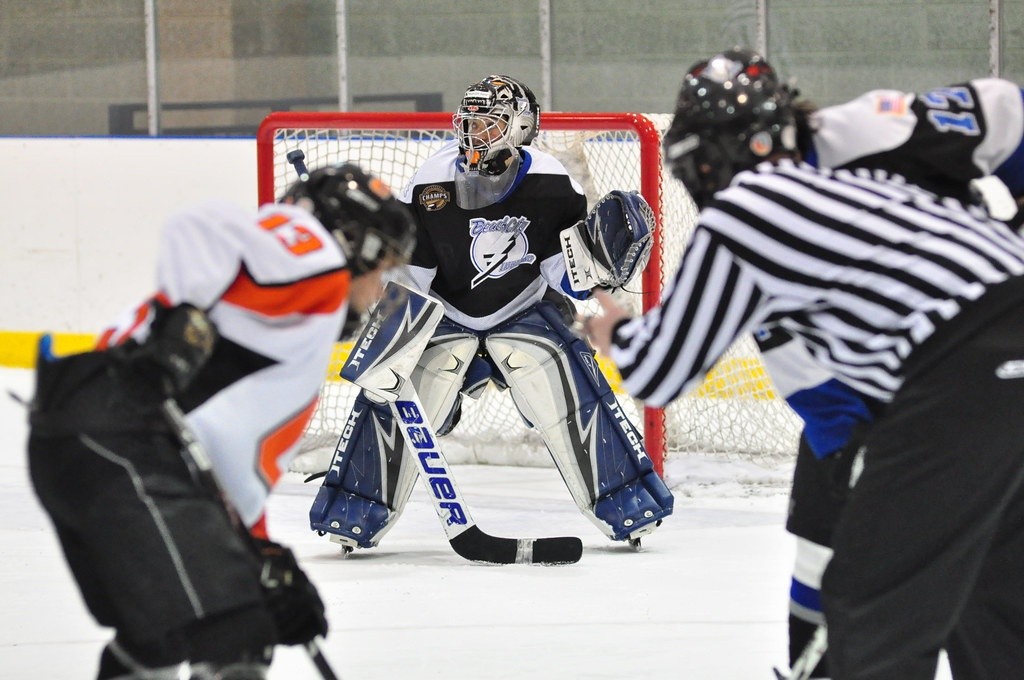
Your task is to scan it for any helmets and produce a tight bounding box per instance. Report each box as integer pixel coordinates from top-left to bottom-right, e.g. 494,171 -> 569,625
453,73 -> 541,146
661,47 -> 812,211
277,164 -> 418,277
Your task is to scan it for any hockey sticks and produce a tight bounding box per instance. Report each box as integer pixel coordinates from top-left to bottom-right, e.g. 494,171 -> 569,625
774,619 -> 826,680
288,149 -> 585,565
157,397 -> 336,680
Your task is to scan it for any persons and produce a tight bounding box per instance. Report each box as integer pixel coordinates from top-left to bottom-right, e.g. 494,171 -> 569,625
29,164 -> 415,680
303,75 -> 673,558
569,46 -> 1024,680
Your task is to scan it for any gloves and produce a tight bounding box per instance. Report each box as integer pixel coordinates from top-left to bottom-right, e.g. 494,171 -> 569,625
257,538 -> 328,645
133,304 -> 218,405
